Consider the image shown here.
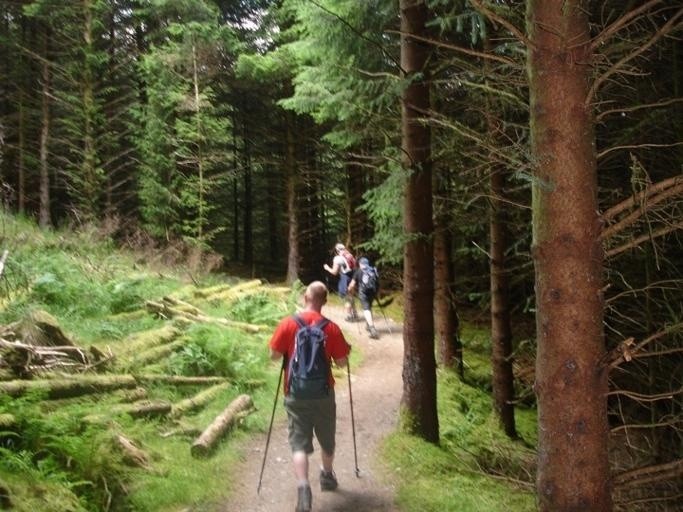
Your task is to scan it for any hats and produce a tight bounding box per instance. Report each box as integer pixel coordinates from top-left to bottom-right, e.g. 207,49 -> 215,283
335,242 -> 345,251
359,257 -> 369,269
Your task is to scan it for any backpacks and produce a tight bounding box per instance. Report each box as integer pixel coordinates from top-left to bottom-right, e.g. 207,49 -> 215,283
359,268 -> 377,296
341,252 -> 358,277
288,315 -> 330,400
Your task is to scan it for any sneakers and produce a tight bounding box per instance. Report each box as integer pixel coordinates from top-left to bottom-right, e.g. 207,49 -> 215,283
367,324 -> 379,339
345,312 -> 360,322
320,471 -> 339,491
296,485 -> 313,512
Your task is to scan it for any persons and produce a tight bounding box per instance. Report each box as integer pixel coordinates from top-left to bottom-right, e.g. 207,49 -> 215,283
348,257 -> 379,338
323,242 -> 359,322
268,280 -> 351,512
